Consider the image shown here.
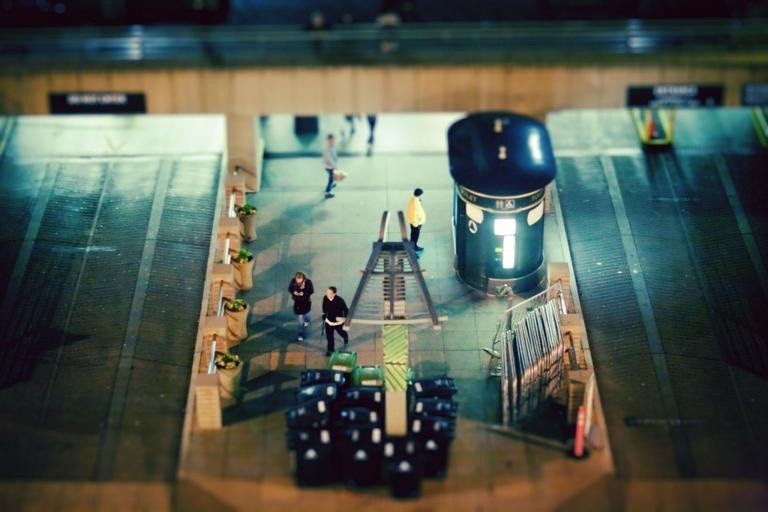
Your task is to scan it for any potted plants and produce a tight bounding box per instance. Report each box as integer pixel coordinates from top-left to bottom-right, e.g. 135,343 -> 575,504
213,203 -> 259,409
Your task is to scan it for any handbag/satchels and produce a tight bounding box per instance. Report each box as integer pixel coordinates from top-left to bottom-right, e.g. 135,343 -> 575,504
333,172 -> 347,182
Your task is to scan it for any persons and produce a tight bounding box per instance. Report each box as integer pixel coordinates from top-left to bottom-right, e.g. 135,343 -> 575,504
406,189 -> 425,250
322,134 -> 339,198
288,272 -> 314,342
322,287 -> 349,356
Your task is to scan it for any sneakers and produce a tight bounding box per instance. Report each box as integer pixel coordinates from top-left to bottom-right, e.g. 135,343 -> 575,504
325,194 -> 334,199
417,247 -> 424,251
326,350 -> 332,357
343,336 -> 349,344
298,335 -> 304,342
304,321 -> 310,326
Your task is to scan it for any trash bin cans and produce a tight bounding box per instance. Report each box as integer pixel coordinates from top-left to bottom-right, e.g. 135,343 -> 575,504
284,350 -> 459,500
